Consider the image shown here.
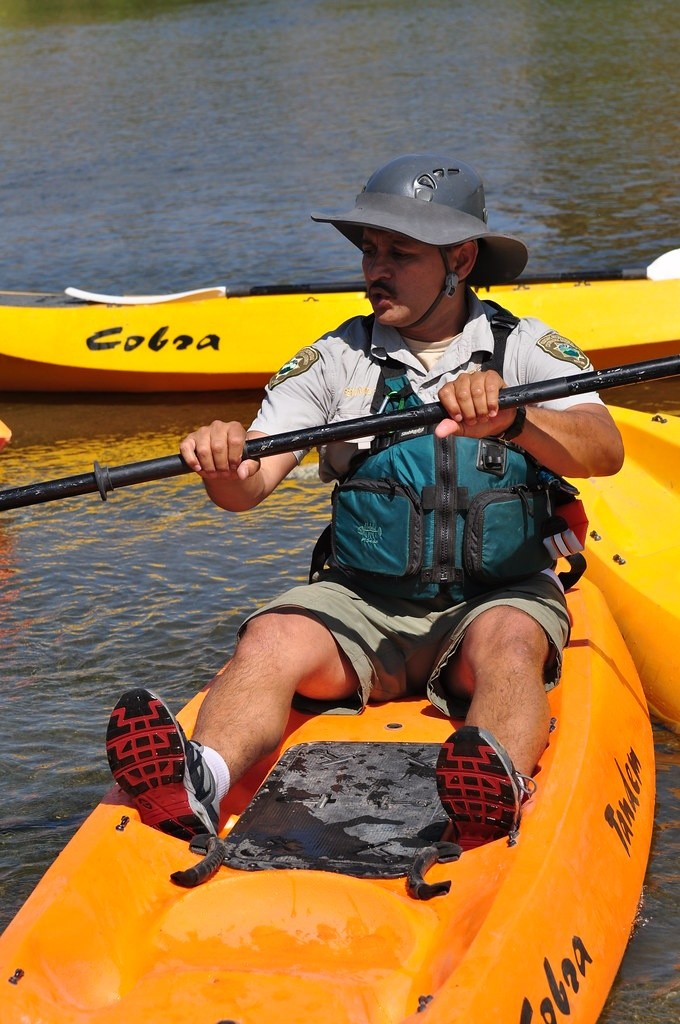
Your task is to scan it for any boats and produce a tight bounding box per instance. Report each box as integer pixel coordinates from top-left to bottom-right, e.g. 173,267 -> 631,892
0,554 -> 655,1024
562,404 -> 679,735
1,267 -> 678,396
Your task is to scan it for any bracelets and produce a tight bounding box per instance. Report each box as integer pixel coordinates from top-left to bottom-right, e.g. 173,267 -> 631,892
492,406 -> 529,440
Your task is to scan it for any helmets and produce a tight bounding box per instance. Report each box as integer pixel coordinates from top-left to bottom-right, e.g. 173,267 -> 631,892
310,155 -> 528,288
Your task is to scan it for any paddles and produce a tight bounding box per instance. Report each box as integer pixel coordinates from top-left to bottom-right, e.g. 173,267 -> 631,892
0,352 -> 680,515
64,247 -> 680,307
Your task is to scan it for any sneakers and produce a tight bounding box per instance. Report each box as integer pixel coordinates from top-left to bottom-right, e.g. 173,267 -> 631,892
106,688 -> 220,843
435,726 -> 537,852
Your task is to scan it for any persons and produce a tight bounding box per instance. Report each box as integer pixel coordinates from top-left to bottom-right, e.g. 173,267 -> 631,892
102,151 -> 624,846
